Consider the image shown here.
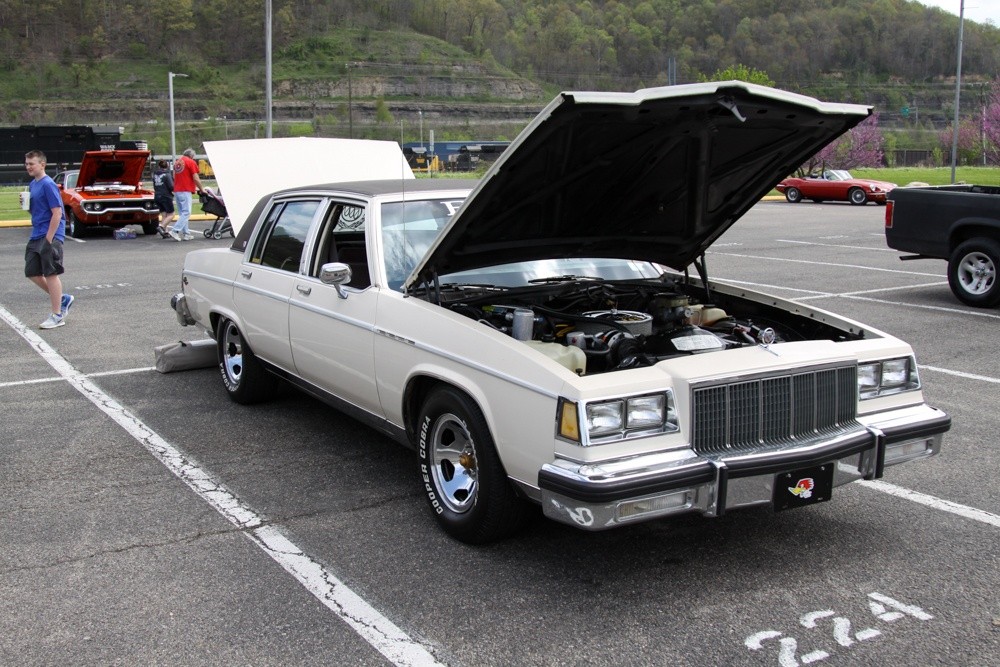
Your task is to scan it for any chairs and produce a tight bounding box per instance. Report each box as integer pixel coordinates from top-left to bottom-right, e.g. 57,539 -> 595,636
335,241 -> 371,289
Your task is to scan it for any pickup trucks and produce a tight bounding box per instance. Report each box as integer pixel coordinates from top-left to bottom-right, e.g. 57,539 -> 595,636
884,183 -> 1000,308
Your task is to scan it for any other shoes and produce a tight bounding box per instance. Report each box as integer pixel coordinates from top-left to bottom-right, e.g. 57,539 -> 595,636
163,231 -> 172,238
156,226 -> 166,239
168,230 -> 182,242
182,233 -> 194,241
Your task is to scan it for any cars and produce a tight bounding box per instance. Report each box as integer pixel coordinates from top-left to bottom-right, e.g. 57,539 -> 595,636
54,149 -> 160,239
171,80 -> 952,548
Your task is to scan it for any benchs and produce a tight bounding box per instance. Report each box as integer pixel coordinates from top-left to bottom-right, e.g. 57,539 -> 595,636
266,230 -> 365,271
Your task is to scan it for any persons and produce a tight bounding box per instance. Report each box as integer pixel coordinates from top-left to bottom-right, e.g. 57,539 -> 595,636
152,160 -> 175,238
18,151 -> 74,329
169,148 -> 208,242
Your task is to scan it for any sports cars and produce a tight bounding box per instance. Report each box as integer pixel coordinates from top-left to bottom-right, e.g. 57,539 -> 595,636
775,169 -> 897,206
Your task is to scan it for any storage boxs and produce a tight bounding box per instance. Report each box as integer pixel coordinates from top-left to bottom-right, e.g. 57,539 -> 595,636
113,228 -> 137,240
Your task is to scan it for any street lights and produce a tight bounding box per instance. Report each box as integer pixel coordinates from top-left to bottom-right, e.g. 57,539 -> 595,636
169,73 -> 191,164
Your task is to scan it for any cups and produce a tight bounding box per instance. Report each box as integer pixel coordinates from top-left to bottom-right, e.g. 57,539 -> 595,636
20,193 -> 30,210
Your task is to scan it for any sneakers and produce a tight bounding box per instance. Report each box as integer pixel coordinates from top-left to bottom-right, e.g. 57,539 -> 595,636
38,313 -> 65,329
61,294 -> 74,319
